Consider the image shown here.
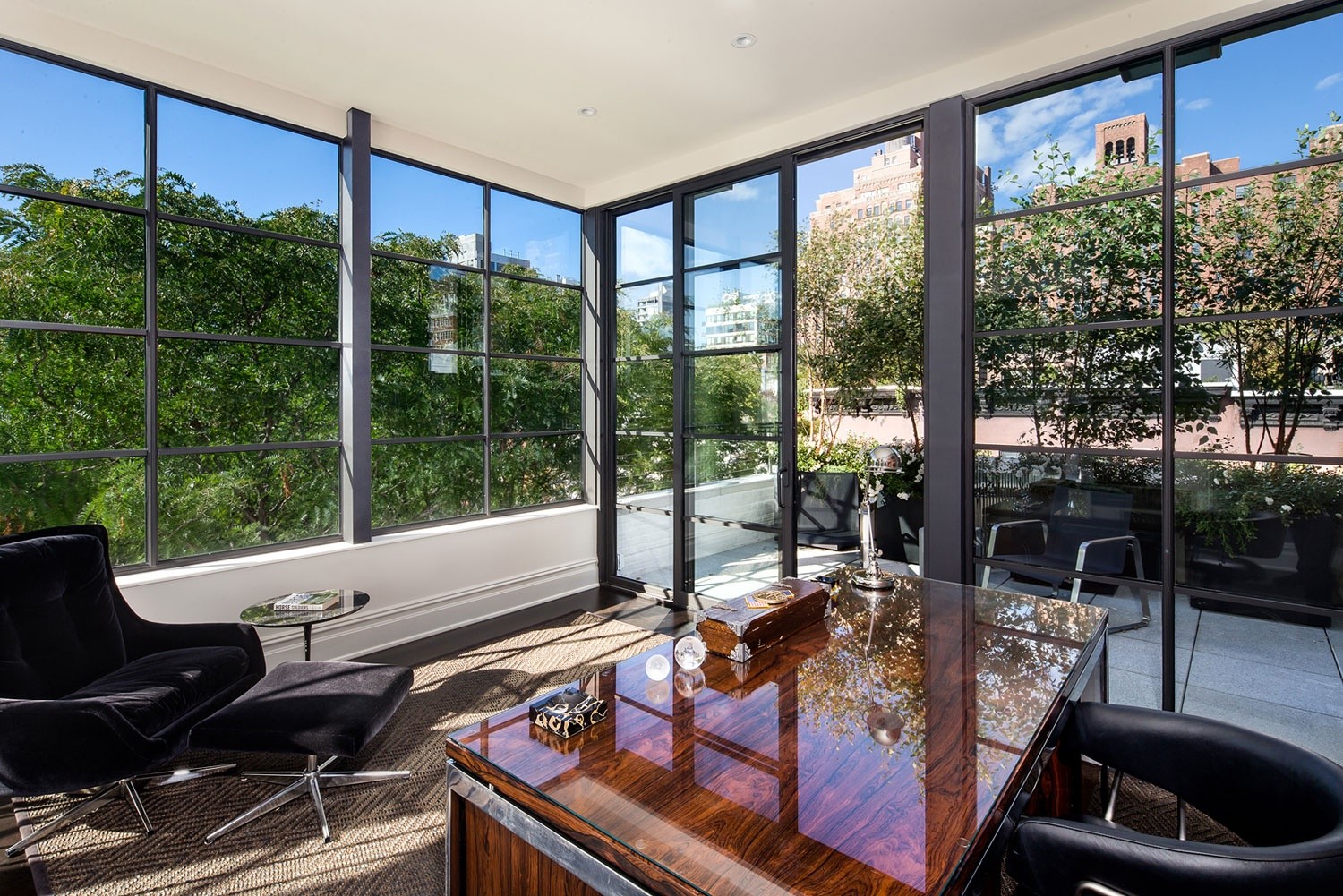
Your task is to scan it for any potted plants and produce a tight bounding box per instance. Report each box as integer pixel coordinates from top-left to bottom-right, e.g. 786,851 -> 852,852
858,448 -> 925,564
1194,477 -> 1292,554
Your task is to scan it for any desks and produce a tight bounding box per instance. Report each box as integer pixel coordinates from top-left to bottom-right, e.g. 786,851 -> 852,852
446,569 -> 1110,896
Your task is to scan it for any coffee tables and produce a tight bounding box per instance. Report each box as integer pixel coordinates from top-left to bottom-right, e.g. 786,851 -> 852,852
241,587 -> 370,661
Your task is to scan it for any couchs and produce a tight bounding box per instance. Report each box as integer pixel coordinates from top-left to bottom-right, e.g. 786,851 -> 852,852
0,522 -> 264,856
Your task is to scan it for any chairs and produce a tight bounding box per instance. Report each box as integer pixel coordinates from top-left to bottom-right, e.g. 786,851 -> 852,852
774,470 -> 861,551
980,485 -> 1150,633
1001,700 -> 1343,896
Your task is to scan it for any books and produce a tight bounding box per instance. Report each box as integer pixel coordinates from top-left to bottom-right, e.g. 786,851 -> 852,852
273,593 -> 340,612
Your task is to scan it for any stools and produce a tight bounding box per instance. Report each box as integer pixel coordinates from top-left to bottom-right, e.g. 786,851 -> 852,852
187,662 -> 415,843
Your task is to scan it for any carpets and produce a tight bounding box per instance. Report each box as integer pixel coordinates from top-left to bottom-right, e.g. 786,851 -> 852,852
11,610 -> 677,895
